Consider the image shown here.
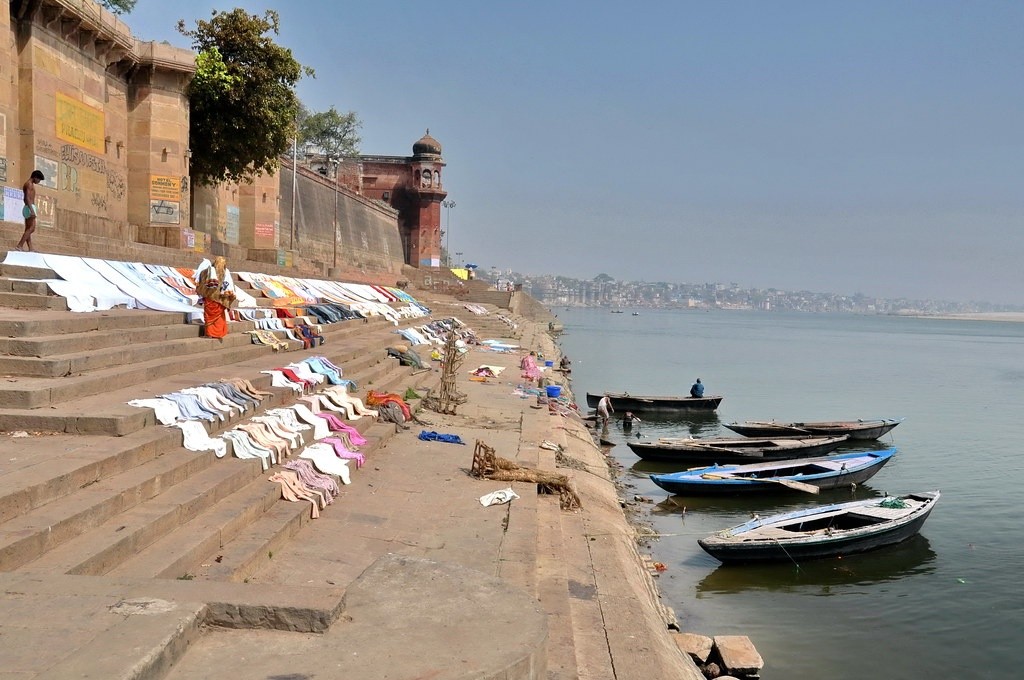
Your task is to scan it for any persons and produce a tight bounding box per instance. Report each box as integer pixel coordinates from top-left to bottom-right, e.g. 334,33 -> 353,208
521,351 -> 540,377
620,408 -> 641,428
690,378 -> 704,398
560,355 -> 570,369
598,395 -> 615,427
15,170 -> 44,253
196,255 -> 235,339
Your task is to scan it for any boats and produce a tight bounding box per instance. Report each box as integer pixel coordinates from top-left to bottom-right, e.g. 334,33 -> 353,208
697,490 -> 942,564
586,392 -> 724,413
611,310 -> 623,313
721,416 -> 905,444
634,312 -> 638,316
626,433 -> 851,467
650,448 -> 898,498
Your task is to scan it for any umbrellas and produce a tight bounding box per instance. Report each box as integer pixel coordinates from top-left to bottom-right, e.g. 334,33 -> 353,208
465,263 -> 477,269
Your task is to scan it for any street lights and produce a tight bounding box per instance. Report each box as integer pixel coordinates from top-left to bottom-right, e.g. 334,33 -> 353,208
443,200 -> 457,267
327,151 -> 343,269
